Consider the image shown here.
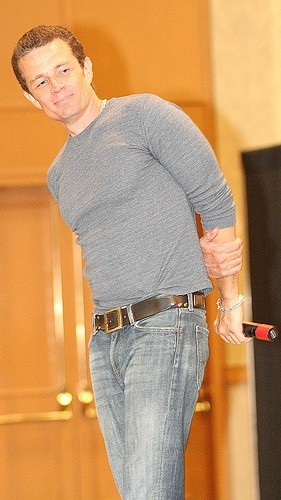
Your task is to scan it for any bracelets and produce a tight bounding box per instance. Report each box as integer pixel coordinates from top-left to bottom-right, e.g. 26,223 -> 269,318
215,294 -> 245,313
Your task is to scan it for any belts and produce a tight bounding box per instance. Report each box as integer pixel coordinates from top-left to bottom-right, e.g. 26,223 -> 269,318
92,291 -> 206,334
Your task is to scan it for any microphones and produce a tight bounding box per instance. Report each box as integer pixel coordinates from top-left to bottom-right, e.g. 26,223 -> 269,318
242,321 -> 279,343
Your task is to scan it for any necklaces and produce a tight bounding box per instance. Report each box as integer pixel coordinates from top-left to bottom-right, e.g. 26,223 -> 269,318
99,97 -> 108,113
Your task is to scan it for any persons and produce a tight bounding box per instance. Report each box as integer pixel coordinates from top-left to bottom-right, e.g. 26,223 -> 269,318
9,24 -> 254,498
